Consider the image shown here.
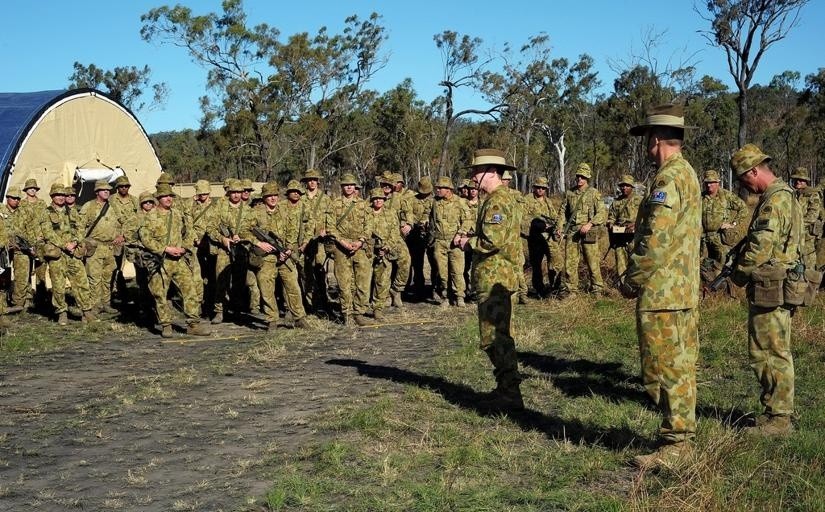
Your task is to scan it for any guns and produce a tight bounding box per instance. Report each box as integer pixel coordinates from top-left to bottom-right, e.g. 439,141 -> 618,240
219,220 -> 243,262
14,236 -> 38,259
559,223 -> 570,245
708,237 -> 747,291
372,233 -> 394,257
252,228 -> 300,264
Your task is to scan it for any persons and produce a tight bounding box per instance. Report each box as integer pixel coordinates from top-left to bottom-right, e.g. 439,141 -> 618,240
616,106 -> 703,470
502,162 -> 643,304
1,170 -> 486,335
702,166 -> 825,311
729,143 -> 805,437
457,149 -> 524,416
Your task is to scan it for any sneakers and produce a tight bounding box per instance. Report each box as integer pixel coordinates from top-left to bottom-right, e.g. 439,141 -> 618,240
58,305 -> 118,326
478,398 -> 524,412
563,291 -> 576,301
630,442 -> 693,469
343,289 -> 403,326
294,320 -> 309,328
432,291 -> 466,308
519,295 -> 529,303
746,413 -> 792,436
266,321 -> 277,334
161,312 -> 223,338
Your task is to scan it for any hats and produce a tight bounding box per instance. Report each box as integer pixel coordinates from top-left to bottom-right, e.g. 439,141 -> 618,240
575,163 -> 592,179
729,143 -> 772,183
533,177 -> 549,189
617,175 -> 635,187
370,171 -> 475,202
193,168 -> 363,195
629,104 -> 700,136
461,149 -> 517,170
789,167 -> 810,180
6,173 -> 175,204
502,170 -> 512,180
703,170 -> 720,182
